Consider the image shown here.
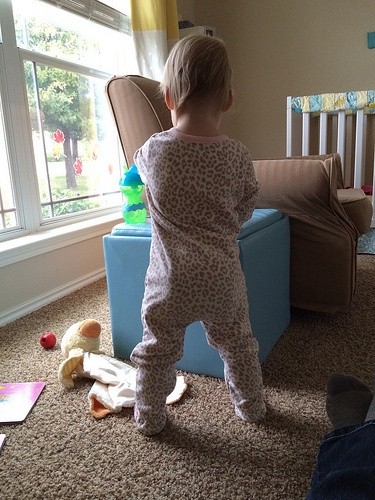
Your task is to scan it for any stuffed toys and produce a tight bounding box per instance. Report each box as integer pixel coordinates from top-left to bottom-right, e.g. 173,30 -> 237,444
57,319 -> 188,418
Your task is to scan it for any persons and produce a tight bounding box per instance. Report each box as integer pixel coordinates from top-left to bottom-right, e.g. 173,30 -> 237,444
129,35 -> 267,437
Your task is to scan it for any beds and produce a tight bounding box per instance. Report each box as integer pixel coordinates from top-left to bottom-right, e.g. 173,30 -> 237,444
285,94 -> 375,254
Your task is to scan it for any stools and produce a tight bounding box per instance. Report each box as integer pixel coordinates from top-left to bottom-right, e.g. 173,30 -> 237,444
103,208 -> 291,379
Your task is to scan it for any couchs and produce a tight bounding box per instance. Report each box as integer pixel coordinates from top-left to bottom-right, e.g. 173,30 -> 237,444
107,74 -> 372,316
